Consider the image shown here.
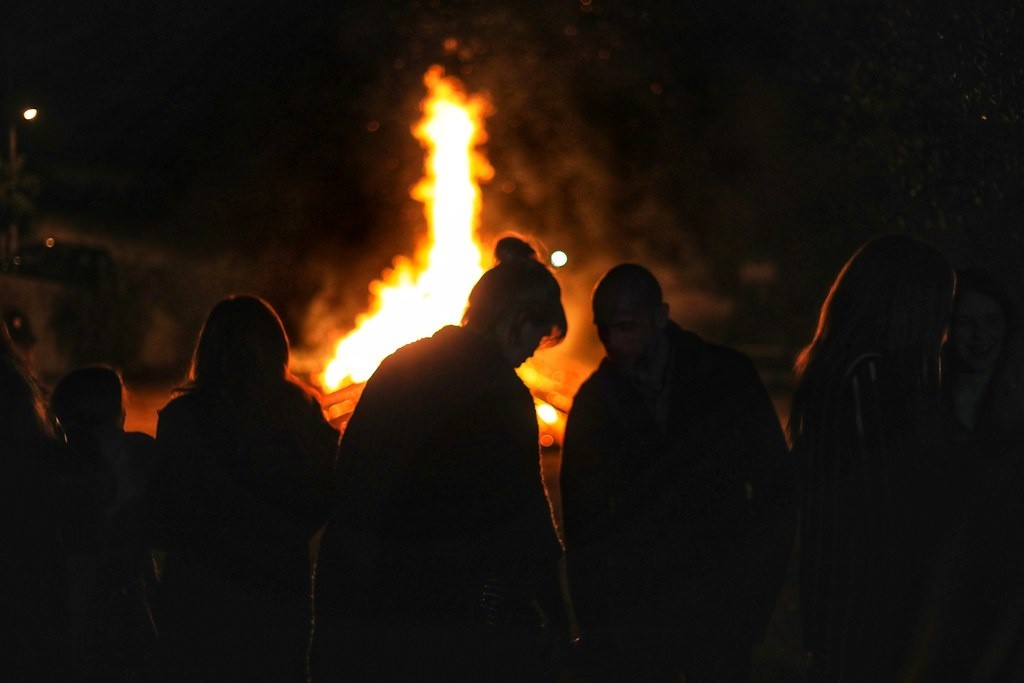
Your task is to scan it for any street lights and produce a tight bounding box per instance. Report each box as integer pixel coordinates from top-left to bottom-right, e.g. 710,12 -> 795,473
8,107 -> 39,255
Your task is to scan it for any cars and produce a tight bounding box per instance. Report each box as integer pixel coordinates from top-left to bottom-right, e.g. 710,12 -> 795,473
0,242 -> 115,296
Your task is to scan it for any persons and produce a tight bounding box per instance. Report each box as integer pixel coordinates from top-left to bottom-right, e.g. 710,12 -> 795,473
559,267 -> 798,683
783,236 -> 1023,683
307,238 -> 581,683
0,293 -> 341,683
